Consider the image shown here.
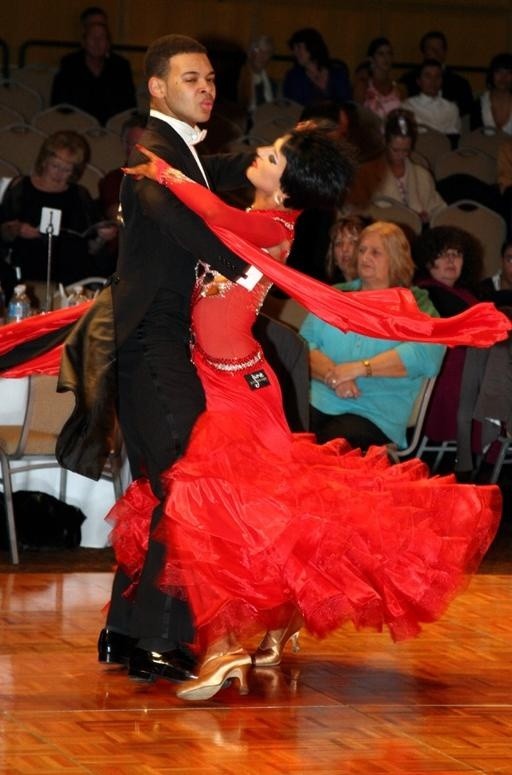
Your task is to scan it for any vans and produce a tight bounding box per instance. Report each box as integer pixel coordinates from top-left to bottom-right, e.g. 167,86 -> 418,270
176,646 -> 252,701
252,609 -> 304,667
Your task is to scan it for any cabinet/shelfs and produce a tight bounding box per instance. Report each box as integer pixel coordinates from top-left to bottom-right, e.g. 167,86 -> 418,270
1,281 -> 29,326
68,286 -> 88,308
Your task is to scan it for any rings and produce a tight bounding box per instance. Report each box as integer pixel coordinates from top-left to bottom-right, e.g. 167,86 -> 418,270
332,380 -> 336,384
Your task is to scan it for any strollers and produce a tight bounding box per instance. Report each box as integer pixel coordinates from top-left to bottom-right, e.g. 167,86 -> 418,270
192,130 -> 207,145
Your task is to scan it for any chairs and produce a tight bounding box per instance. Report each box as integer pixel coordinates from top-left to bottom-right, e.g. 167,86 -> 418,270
0,301 -> 127,566
356,58 -> 511,276
1,52 -> 140,197
225,96 -> 307,148
45,276 -> 114,311
375,325 -> 512,494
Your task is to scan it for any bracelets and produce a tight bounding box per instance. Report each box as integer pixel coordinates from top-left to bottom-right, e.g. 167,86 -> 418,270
362,359 -> 372,377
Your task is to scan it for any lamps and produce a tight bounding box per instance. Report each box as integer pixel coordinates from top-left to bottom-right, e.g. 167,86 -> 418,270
45,276 -> 114,311
0,301 -> 127,566
375,325 -> 512,494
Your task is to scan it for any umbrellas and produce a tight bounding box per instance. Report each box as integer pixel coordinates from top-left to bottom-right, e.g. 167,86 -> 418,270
98,628 -> 136,665
127,646 -> 232,688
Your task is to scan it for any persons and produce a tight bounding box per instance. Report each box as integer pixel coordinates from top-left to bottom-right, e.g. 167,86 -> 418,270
299,102 -> 506,454
48,6 -> 138,126
243,27 -> 509,150
1,112 -> 149,307
122,120 -> 511,703
96,33 -> 291,685
